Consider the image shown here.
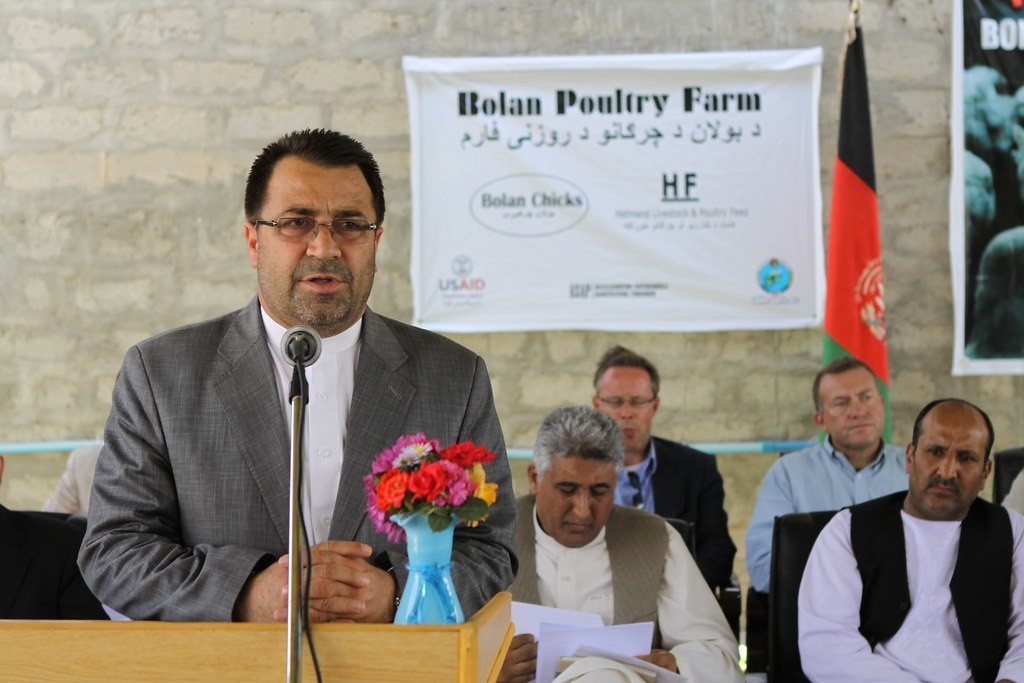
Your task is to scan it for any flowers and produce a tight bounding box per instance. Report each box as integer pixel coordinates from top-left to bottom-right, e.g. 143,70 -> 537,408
363,431 -> 500,543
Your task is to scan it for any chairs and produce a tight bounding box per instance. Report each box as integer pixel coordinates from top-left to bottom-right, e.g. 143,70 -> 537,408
766,507 -> 839,683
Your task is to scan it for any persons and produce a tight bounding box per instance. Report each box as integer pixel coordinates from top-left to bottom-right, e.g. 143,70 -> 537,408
76,127 -> 522,624
512,404 -> 744,681
591,344 -> 739,603
742,359 -> 908,597
796,397 -> 1023,683
0,440 -> 119,620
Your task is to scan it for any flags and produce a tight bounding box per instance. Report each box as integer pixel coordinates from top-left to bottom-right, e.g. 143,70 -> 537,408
821,0 -> 893,441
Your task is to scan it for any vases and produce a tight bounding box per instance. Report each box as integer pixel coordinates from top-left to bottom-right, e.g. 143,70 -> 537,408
388,510 -> 467,625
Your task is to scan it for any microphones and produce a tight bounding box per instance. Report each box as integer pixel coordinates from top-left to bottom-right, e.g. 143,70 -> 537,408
281,324 -> 322,367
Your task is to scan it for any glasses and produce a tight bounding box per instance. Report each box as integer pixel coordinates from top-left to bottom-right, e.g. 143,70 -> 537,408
597,395 -> 655,406
250,217 -> 377,244
